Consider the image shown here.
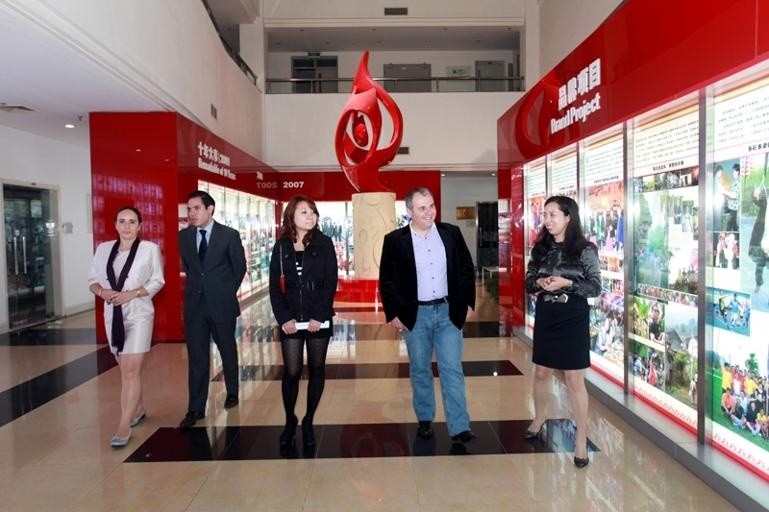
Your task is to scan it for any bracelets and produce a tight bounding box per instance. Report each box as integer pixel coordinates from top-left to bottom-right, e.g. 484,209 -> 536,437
136,289 -> 141,298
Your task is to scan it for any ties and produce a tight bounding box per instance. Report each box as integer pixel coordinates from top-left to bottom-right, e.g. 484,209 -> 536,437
197,228 -> 208,267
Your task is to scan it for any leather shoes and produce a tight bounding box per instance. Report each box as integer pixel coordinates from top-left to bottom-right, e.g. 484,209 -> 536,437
418,421 -> 433,439
452,431 -> 479,443
130,410 -> 147,429
179,410 -> 205,424
225,396 -> 239,410
111,428 -> 132,449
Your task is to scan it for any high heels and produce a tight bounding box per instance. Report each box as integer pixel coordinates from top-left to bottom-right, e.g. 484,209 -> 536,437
572,442 -> 590,468
525,421 -> 544,440
280,417 -> 297,446
302,420 -> 316,450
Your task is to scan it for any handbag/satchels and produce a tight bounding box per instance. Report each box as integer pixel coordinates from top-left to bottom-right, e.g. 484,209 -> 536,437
278,238 -> 287,298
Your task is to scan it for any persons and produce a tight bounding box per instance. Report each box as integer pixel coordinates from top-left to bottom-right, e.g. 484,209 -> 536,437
178,190 -> 248,430
707,163 -> 741,233
596,312 -> 624,363
524,196 -> 602,460
626,337 -> 666,391
706,288 -> 750,336
688,374 -> 698,405
268,195 -> 338,448
706,231 -> 739,269
720,361 -> 769,440
584,204 -> 626,252
87,206 -> 165,449
378,187 -> 482,443
632,295 -> 668,345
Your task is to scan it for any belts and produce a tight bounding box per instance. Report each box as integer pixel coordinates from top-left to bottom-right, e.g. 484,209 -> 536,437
416,297 -> 451,306
537,292 -> 586,303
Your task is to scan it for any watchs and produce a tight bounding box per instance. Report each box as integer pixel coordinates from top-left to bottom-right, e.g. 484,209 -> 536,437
98,287 -> 103,298
